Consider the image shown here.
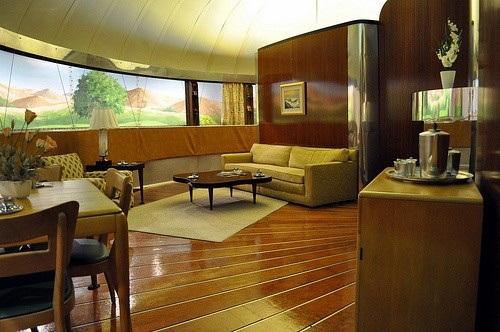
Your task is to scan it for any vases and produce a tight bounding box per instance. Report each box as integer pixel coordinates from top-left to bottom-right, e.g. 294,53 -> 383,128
440,70 -> 456,88
0,177 -> 34,200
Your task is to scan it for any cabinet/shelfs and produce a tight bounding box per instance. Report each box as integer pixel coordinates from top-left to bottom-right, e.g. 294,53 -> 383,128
356,197 -> 484,332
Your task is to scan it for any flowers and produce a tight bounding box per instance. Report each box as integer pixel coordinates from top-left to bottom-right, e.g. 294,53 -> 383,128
436,19 -> 467,70
0,111 -> 54,179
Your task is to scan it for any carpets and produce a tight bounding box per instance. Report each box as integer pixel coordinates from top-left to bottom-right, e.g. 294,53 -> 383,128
121,187 -> 289,243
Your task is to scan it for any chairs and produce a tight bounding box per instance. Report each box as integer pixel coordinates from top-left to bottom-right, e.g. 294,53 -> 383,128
0,162 -> 133,332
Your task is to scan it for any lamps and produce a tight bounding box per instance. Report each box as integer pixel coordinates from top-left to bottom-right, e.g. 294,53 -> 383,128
87,109 -> 121,162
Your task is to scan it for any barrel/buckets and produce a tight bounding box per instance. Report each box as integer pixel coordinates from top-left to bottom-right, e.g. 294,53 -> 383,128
418,121 -> 450,179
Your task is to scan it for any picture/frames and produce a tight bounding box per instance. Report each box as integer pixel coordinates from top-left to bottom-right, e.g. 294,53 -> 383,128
280,82 -> 305,116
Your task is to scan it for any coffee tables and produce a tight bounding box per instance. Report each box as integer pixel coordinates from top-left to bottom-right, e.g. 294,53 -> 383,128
173,171 -> 273,211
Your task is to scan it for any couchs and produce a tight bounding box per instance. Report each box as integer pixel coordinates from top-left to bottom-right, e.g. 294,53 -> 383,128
220,143 -> 359,208
39,152 -> 134,209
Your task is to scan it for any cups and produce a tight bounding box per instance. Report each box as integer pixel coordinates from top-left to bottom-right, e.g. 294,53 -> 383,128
393,157 -> 417,176
447,147 -> 461,176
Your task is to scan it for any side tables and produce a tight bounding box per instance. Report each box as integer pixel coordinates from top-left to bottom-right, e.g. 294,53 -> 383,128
85,162 -> 145,205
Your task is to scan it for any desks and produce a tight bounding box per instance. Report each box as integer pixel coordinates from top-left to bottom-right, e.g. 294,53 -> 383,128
0,178 -> 132,332
